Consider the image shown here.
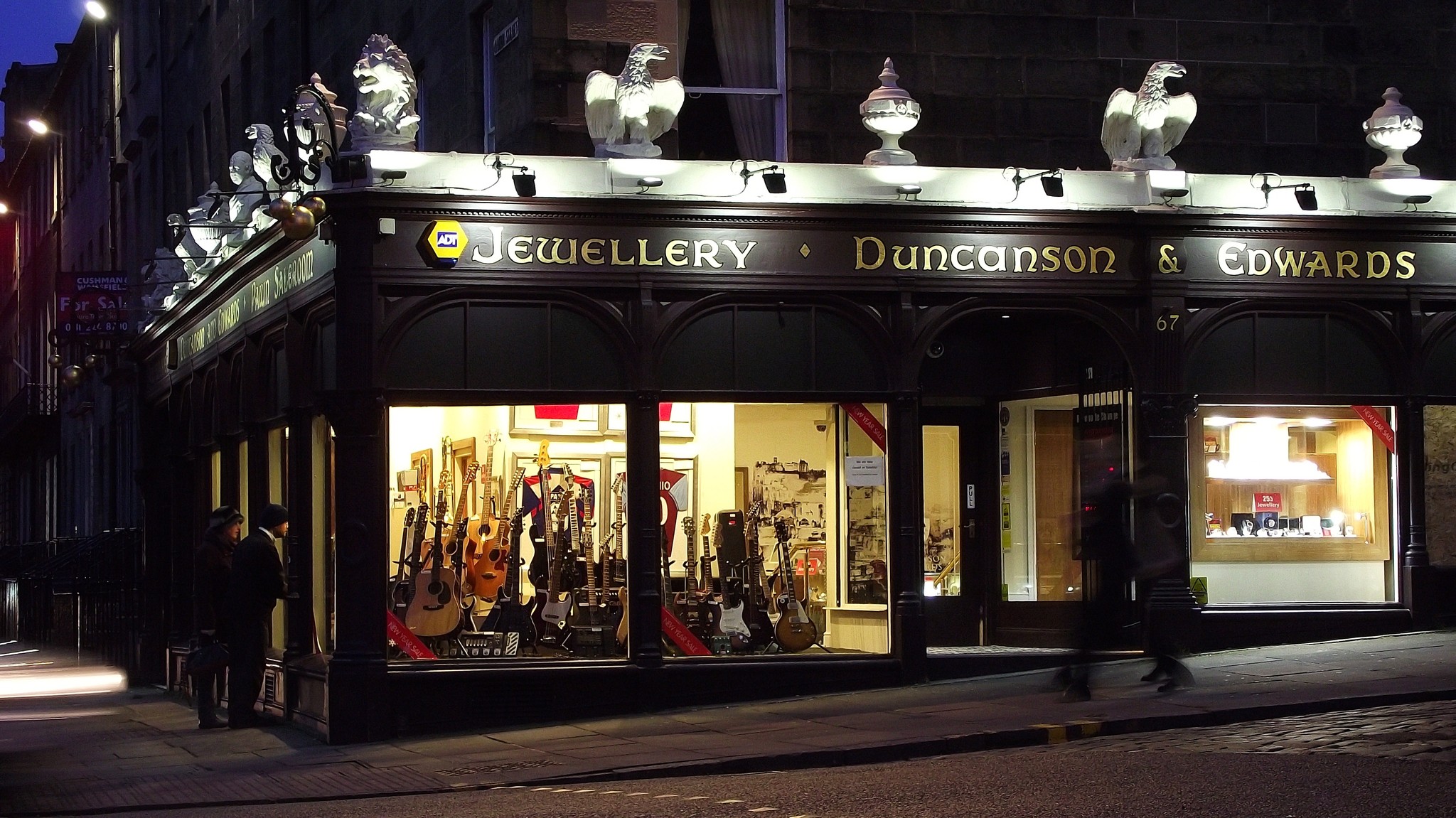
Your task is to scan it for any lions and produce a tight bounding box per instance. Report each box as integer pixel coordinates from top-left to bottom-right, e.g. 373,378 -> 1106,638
348,34 -> 421,153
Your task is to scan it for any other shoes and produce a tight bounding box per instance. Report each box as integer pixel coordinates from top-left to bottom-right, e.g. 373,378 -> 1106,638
199,720 -> 228,729
228,715 -> 279,729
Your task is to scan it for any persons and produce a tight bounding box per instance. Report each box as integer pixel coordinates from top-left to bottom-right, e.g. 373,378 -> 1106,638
190,506 -> 247,729
225,499 -> 297,728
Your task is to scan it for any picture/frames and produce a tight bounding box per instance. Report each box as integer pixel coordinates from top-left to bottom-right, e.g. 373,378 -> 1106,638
603,403 -> 696,438
511,451 -> 605,572
604,452 -> 699,578
509,405 -> 604,437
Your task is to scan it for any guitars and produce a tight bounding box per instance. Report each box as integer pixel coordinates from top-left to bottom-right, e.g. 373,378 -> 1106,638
387,429 -> 834,656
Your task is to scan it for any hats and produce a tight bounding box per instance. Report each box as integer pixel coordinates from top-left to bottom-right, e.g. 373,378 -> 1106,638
257,503 -> 289,527
206,506 -> 245,532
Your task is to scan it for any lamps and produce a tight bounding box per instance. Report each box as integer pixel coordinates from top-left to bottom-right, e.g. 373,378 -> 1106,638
383,157 -> 1447,221
1355,511 -> 1369,544
85,174 -> 301,350
